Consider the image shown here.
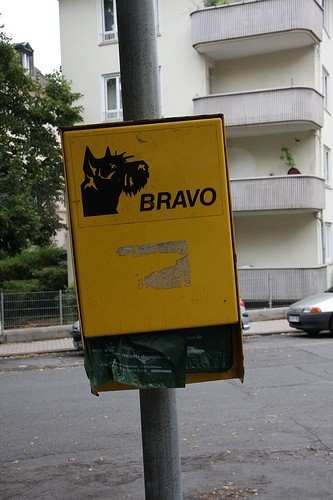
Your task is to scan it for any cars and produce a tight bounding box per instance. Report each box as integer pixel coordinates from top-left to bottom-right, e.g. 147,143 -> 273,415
287,288 -> 332,336
69,298 -> 250,349
